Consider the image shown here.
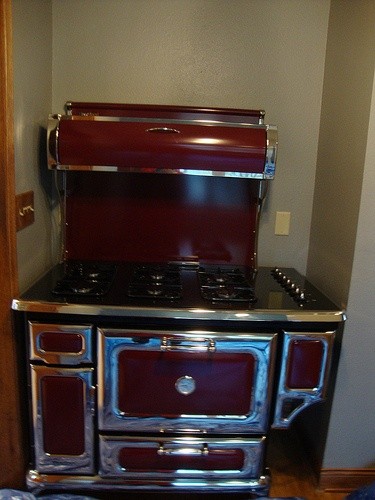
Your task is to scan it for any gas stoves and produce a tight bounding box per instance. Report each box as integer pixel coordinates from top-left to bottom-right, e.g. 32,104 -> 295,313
11,259 -> 346,322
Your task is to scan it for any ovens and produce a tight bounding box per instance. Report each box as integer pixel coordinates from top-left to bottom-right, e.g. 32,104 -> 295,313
99,330 -> 278,481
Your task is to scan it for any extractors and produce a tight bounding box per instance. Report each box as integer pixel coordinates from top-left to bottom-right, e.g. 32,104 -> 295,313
46,101 -> 278,268
